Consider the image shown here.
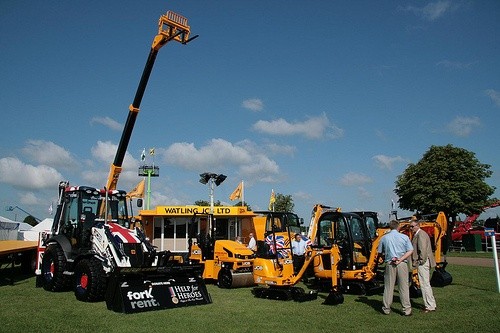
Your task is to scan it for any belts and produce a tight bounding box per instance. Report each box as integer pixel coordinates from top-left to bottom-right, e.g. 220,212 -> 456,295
294,254 -> 304,257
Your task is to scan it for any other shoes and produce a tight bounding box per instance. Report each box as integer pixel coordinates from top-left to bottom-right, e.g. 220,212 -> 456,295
419,308 -> 437,313
300,279 -> 304,281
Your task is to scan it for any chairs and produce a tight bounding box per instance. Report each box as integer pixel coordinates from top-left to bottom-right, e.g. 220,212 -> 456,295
82,207 -> 92,217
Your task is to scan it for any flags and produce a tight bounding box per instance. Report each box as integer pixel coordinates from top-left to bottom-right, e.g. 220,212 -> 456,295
141,149 -> 145,161
150,149 -> 154,155
230,181 -> 243,202
127,181 -> 144,199
269,192 -> 275,210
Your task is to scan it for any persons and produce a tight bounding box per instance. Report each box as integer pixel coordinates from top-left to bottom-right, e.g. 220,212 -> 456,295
378,221 -> 415,316
246,233 -> 256,254
410,220 -> 437,313
291,234 -> 308,282
235,236 -> 242,245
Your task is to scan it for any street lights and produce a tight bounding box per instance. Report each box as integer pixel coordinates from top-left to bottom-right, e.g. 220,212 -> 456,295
198,172 -> 227,247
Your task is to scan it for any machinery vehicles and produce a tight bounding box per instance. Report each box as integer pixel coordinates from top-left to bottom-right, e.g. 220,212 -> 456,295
95,10 -> 500,307
34,179 -> 214,313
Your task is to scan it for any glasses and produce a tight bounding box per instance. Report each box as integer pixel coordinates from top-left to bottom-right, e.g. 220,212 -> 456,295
410,226 -> 414,228
